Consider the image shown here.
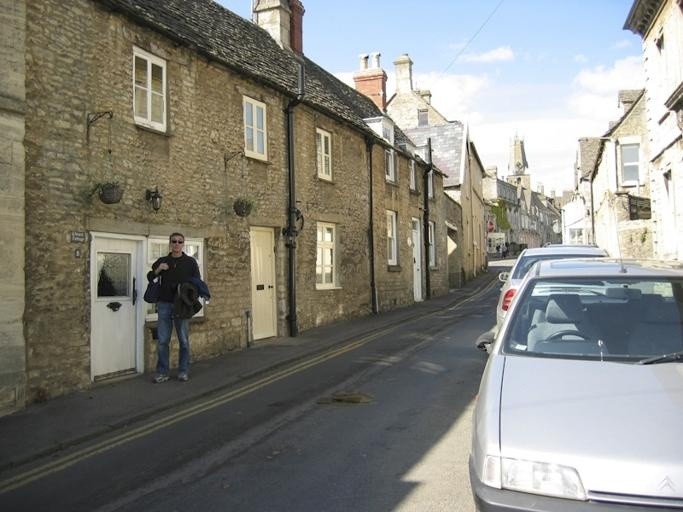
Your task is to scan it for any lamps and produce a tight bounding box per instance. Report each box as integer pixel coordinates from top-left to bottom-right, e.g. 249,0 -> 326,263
146,186 -> 162,214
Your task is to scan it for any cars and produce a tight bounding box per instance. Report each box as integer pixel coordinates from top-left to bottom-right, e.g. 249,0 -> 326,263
491,242 -> 610,332
466,256 -> 683,512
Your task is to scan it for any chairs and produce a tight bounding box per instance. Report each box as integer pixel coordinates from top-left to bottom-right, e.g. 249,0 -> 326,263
526,294 -> 602,351
626,304 -> 683,354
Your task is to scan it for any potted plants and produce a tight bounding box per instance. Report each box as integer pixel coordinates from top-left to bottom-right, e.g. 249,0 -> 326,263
87,181 -> 124,203
232,198 -> 253,216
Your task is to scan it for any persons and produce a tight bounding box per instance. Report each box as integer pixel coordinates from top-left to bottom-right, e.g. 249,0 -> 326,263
147,232 -> 201,383
501,244 -> 507,259
496,243 -> 500,257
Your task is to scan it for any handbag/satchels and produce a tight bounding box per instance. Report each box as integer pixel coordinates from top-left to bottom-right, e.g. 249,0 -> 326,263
144,272 -> 162,303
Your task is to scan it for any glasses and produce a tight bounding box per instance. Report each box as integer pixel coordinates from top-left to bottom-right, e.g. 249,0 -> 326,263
172,239 -> 184,244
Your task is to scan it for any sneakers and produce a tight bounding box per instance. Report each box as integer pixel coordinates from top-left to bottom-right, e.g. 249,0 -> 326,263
178,373 -> 188,381
153,374 -> 170,383
